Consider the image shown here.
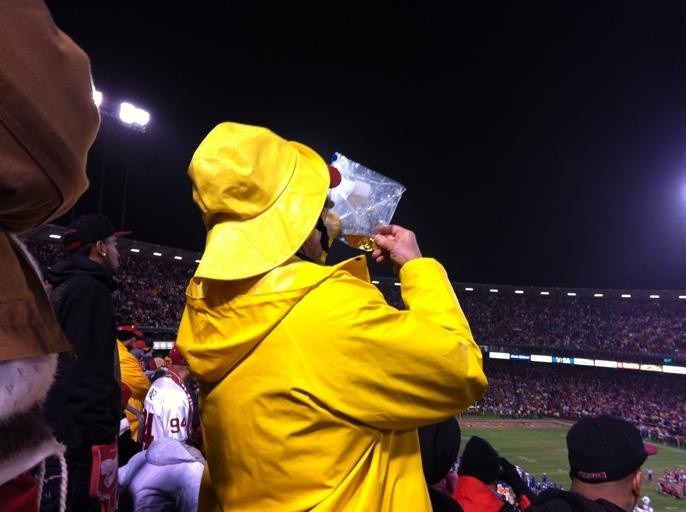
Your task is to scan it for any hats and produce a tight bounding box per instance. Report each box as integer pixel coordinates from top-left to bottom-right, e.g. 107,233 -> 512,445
187,122 -> 330,280
63,212 -> 132,251
117,325 -> 144,337
169,344 -> 188,366
458,435 -> 499,485
419,417 -> 461,484
566,416 -> 657,484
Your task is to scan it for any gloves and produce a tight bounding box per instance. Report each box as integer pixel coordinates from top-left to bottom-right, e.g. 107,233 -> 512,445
499,457 -> 536,504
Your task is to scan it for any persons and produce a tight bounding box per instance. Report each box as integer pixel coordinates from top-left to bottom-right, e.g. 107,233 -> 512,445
173,120 -> 489,507
378,286 -> 685,512
1,222 -> 206,512
0,0 -> 102,469
